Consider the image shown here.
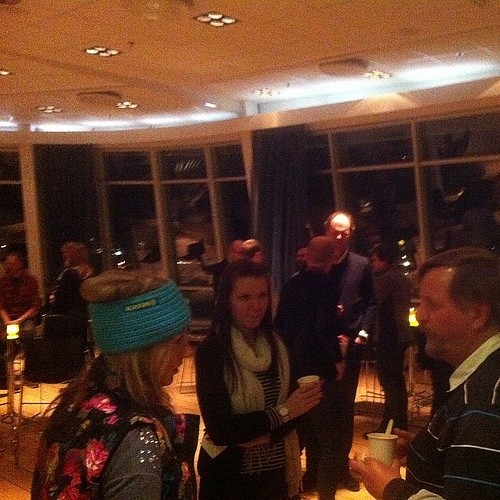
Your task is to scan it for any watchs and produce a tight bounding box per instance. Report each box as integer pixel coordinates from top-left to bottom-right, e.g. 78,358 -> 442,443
275,403 -> 290,424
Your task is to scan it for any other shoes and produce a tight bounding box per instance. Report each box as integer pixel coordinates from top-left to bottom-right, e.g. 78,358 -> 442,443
298,470 -> 316,493
342,472 -> 360,491
362,428 -> 395,439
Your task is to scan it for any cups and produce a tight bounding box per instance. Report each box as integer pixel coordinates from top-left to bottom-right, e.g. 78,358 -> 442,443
367,432 -> 399,468
297,375 -> 319,387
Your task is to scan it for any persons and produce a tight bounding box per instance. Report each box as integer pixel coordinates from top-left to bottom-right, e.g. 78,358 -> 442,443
194,237 -> 323,500
345,245 -> 500,500
0,238 -> 94,345
29,266 -> 198,500
277,210 -> 411,500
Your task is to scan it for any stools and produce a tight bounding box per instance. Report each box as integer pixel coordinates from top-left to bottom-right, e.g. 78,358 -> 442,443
179,286 -> 216,392
12,314 -> 86,468
